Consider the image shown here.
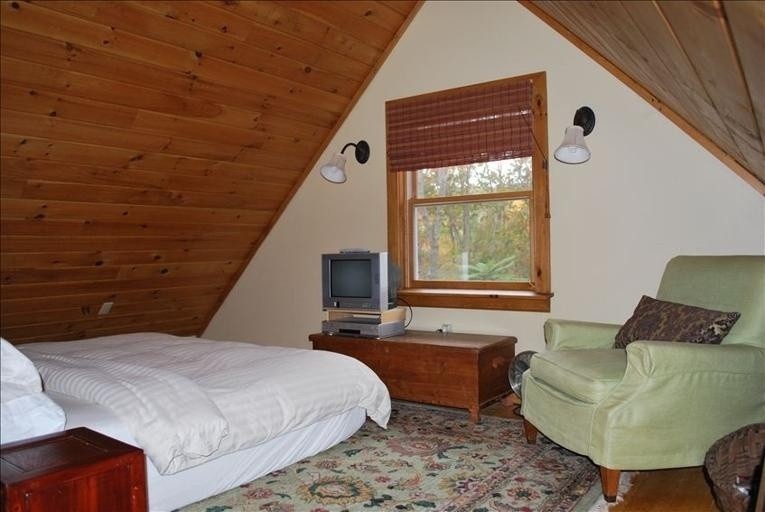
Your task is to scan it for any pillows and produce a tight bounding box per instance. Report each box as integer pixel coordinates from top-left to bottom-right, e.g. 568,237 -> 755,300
613,294 -> 741,352
0,333 -> 67,431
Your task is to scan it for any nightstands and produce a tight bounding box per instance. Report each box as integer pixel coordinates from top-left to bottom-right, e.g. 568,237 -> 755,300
0,424 -> 151,510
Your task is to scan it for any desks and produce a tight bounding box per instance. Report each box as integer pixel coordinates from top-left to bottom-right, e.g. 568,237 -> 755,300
309,328 -> 518,424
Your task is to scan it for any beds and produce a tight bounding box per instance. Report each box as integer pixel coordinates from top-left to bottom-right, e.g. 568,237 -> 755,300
0,327 -> 368,511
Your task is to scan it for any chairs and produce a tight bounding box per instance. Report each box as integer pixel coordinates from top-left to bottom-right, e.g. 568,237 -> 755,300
522,253 -> 765,502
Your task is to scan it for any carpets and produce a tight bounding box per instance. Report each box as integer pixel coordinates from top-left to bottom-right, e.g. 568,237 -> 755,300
185,402 -> 643,512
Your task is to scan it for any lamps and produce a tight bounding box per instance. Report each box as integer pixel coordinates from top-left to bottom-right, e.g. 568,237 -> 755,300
553,105 -> 596,166
320,138 -> 371,184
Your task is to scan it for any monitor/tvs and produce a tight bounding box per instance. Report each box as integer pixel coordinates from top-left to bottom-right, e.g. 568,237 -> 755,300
322,252 -> 400,311
